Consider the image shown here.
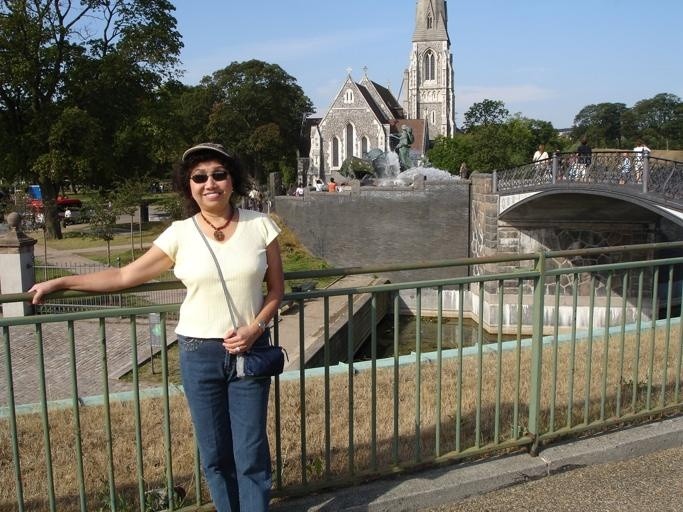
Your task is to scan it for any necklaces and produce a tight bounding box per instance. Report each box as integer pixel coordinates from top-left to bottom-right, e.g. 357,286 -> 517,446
200,204 -> 235,241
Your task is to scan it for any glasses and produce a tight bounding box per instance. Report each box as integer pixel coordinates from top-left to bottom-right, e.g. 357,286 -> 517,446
188,171 -> 228,183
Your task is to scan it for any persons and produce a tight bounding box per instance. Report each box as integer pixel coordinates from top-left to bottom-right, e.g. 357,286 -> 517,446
618,152 -> 632,185
28,143 -> 285,511
249,187 -> 263,211
21,206 -> 45,230
531,137 -> 592,183
64,207 -> 72,226
633,140 -> 652,183
388,124 -> 414,149
287,178 -> 346,197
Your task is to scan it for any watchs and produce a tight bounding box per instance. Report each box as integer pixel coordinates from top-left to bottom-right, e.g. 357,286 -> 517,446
254,320 -> 266,333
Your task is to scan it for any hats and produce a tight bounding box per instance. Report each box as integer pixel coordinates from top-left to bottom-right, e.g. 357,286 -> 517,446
182,142 -> 231,161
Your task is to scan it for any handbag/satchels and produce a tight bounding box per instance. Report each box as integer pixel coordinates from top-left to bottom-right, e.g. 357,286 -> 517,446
236,346 -> 284,378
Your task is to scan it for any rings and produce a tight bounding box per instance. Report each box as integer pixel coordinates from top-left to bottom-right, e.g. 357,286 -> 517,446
236,346 -> 241,353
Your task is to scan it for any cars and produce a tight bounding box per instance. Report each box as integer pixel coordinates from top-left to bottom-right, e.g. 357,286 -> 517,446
27,193 -> 83,213
